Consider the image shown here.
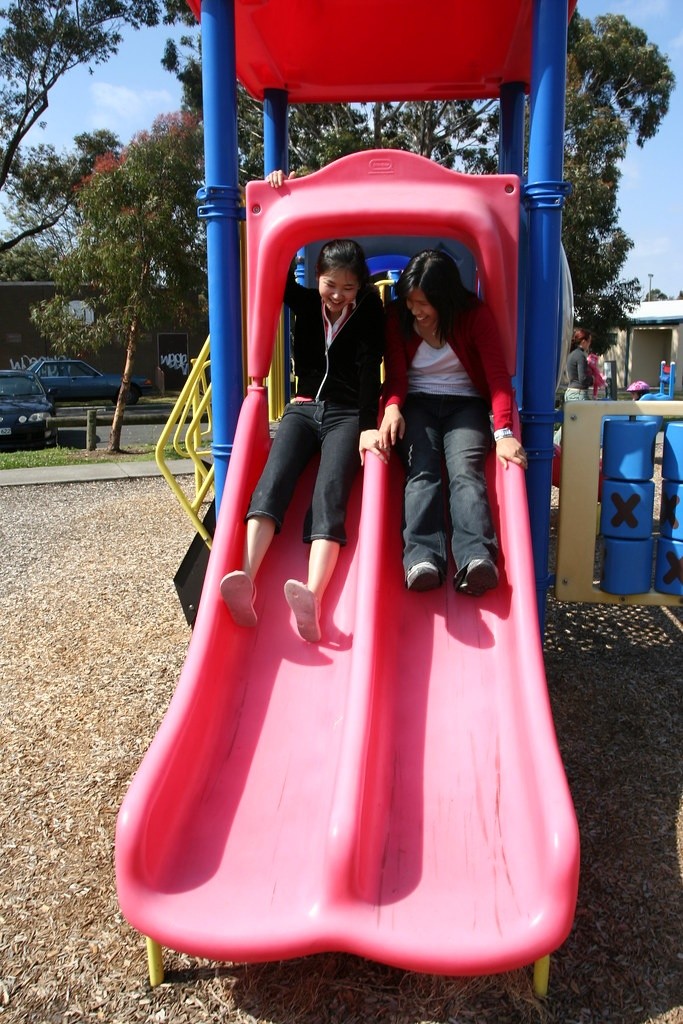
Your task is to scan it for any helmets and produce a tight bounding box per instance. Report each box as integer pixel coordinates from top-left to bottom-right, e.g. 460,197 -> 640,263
627,381 -> 650,392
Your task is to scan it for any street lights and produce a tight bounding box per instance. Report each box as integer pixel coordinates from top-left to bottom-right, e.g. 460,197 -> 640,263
648,274 -> 654,301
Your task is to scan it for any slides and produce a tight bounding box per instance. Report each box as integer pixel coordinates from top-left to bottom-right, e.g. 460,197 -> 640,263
113,183 -> 580,975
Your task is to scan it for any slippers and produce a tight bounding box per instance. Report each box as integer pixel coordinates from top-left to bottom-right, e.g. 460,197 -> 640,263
285,579 -> 321,643
219,570 -> 258,628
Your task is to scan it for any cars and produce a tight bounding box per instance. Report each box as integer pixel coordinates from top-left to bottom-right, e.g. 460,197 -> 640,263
0,369 -> 59,452
23,359 -> 153,405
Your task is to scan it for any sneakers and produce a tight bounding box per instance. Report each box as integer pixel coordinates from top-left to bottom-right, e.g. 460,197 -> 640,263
407,563 -> 441,592
459,558 -> 499,597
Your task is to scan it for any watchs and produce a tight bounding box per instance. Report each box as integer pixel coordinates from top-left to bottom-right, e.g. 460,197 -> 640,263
493,427 -> 513,442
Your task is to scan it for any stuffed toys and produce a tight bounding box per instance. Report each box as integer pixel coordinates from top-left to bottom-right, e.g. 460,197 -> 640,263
586,353 -> 608,399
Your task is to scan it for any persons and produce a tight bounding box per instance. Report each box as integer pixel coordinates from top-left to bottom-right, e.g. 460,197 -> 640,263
552,328 -> 595,446
221,236 -> 387,647
624,380 -> 651,403
373,250 -> 516,596
